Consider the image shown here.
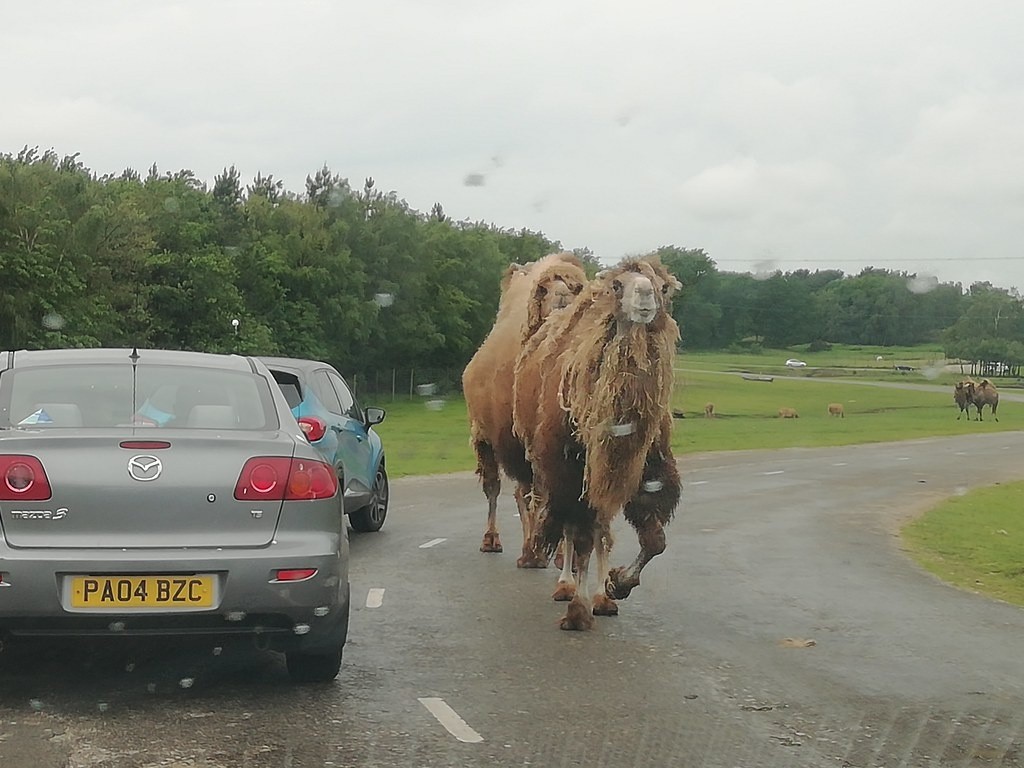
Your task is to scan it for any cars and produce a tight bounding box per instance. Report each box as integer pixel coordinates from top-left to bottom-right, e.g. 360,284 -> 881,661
127,353 -> 390,532
987,362 -> 1010,375
786,359 -> 807,368
895,364 -> 916,371
0,348 -> 350,680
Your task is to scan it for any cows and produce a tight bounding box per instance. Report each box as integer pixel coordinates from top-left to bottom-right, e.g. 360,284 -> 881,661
704,403 -> 713,418
828,404 -> 844,418
779,408 -> 799,420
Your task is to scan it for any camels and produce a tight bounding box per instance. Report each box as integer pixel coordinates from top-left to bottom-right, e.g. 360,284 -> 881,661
462,251 -> 587,572
512,253 -> 684,629
953,375 -> 999,422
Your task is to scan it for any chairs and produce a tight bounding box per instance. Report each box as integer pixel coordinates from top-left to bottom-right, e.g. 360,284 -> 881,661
187,404 -> 240,428
30,403 -> 85,428
